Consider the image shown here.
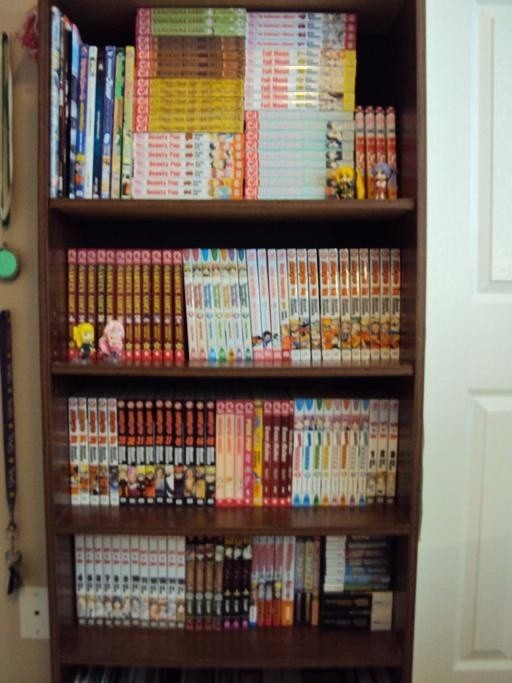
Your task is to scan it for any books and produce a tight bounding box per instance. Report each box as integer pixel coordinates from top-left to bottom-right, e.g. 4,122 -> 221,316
67,246 -> 401,363
70,399 -> 403,505
74,535 -> 396,633
47,6 -> 399,200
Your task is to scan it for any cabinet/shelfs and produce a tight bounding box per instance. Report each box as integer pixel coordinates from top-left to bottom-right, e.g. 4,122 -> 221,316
37,1 -> 428,683
